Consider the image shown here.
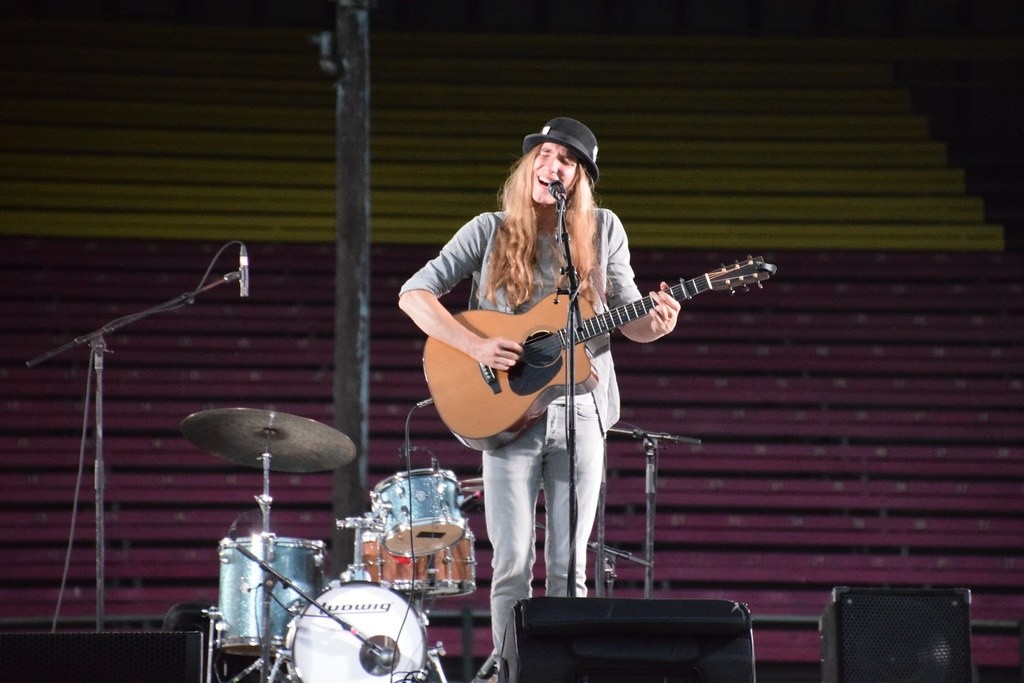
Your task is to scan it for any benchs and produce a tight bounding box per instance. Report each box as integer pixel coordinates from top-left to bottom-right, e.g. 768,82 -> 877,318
3,14 -> 1021,682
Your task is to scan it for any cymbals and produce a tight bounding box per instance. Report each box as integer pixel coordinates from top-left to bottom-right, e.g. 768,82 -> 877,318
179,407 -> 357,474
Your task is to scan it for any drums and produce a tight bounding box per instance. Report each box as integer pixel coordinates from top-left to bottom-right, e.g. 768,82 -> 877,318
285,571 -> 430,683
369,466 -> 470,558
361,530 -> 477,601
217,536 -> 328,657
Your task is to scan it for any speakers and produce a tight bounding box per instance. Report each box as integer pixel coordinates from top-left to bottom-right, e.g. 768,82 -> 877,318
496,597 -> 756,683
818,587 -> 973,683
0,632 -> 203,683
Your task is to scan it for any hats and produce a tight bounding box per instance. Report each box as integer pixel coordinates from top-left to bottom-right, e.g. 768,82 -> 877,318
521,116 -> 602,182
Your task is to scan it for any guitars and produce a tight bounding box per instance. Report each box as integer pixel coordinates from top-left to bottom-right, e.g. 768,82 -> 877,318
422,253 -> 778,452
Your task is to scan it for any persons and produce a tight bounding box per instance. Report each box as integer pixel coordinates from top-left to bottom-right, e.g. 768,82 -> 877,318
398,117 -> 682,683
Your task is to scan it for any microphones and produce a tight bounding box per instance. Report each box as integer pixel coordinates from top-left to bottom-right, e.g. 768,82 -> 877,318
239,244 -> 249,298
548,180 -> 567,200
343,623 -> 397,669
